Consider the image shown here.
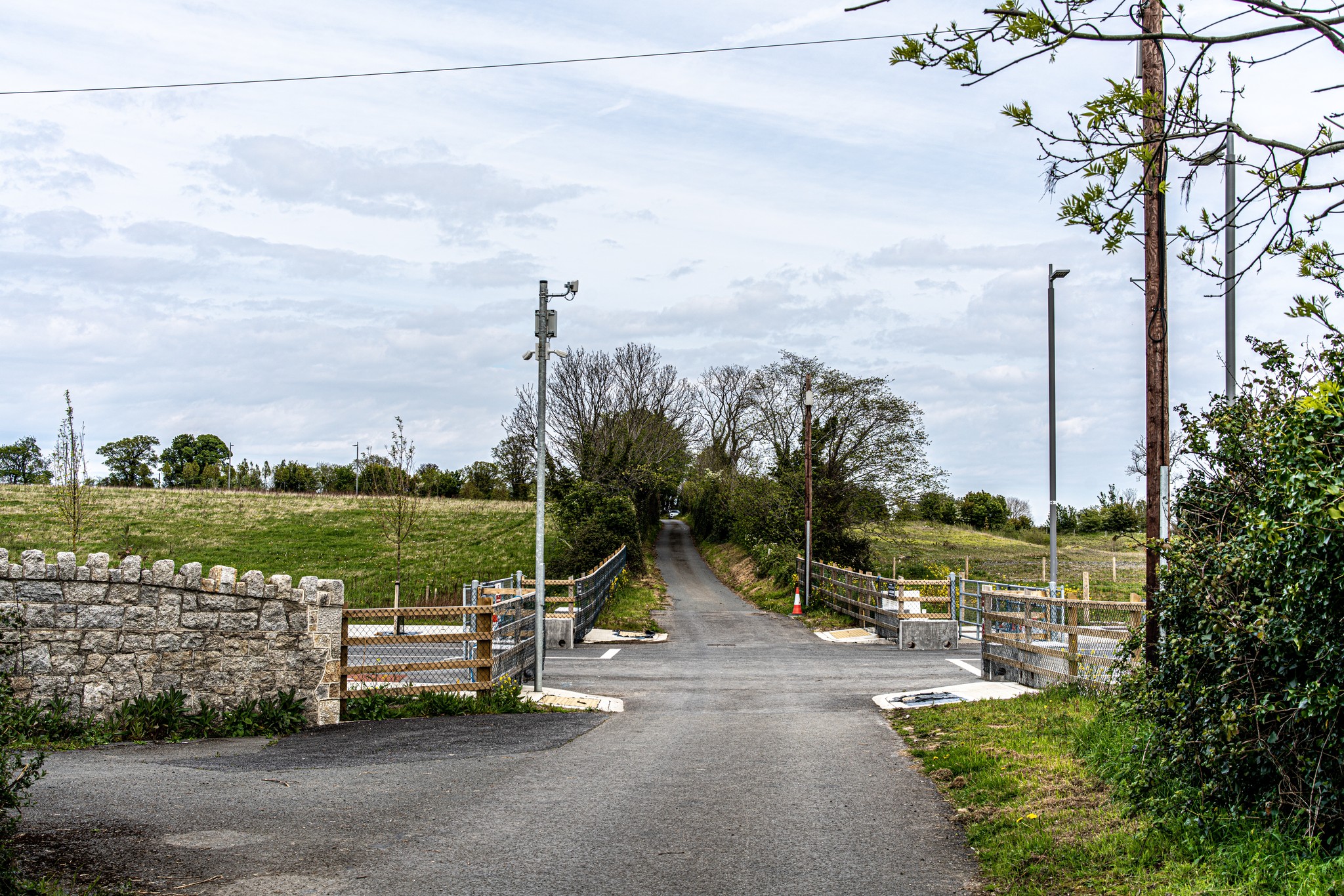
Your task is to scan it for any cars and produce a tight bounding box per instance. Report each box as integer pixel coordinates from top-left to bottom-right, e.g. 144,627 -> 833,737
669,510 -> 680,519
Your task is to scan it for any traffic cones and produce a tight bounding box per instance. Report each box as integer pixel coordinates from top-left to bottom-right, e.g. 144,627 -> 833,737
787,586 -> 806,618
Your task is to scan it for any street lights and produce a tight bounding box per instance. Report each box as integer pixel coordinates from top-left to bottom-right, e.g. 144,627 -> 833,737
803,374 -> 816,612
228,442 -> 234,490
1046,262 -> 1071,645
1188,119 -> 1239,417
352,442 -> 359,496
522,278 -> 579,697
